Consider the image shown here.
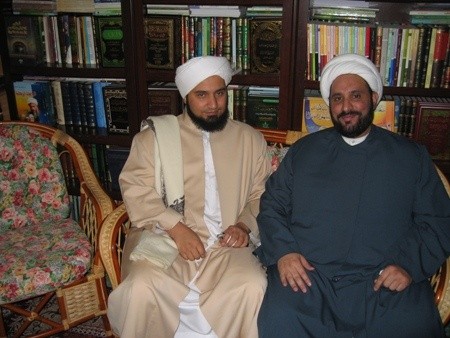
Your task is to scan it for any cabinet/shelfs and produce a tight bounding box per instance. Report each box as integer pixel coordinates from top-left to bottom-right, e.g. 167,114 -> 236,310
0,0 -> 450,220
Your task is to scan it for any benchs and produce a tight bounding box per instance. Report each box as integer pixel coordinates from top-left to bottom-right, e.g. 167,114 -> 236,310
95,126 -> 450,324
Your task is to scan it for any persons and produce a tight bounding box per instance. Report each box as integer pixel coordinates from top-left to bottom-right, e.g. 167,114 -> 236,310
108,55 -> 273,338
251,54 -> 449,338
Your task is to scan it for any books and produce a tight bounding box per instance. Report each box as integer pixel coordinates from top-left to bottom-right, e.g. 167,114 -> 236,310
302,90 -> 450,158
57,144 -> 131,192
13,78 -> 130,134
306,0 -> 450,89
4,1 -> 126,68
148,81 -> 279,130
145,4 -> 282,74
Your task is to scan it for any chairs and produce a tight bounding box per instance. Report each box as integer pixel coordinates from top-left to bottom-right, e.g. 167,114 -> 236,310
0,120 -> 117,338
